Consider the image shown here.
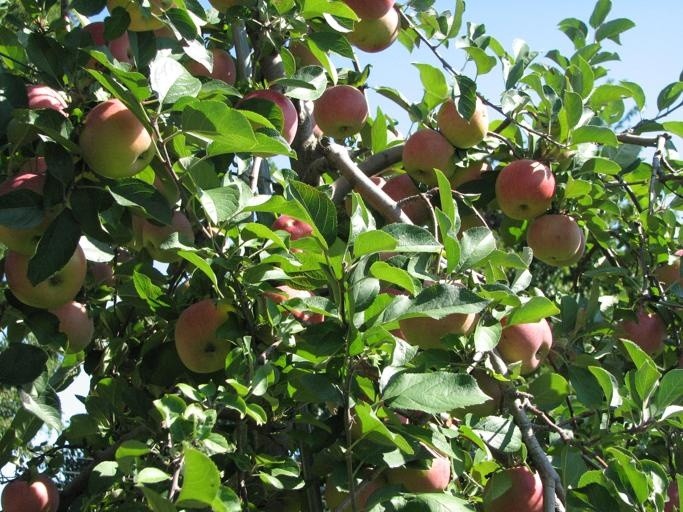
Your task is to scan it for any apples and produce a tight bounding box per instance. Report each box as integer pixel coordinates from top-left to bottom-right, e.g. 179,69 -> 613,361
2,1 -> 682,511
